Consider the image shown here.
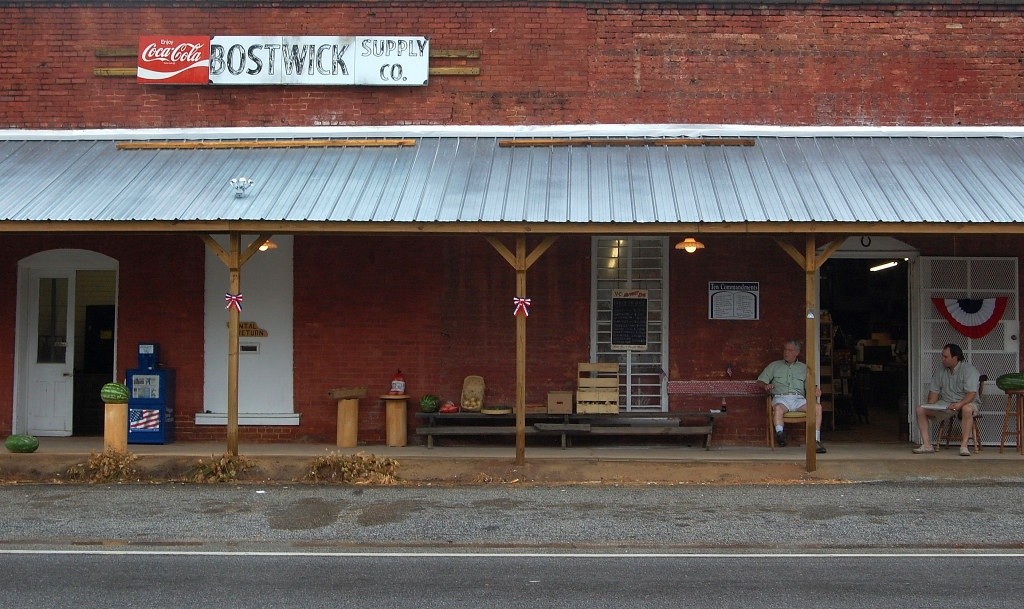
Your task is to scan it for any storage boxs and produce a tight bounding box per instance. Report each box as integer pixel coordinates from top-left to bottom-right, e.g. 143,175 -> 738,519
547,391 -> 572,414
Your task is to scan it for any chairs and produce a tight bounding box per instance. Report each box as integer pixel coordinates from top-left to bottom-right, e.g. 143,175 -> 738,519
936,375 -> 988,452
766,385 -> 818,451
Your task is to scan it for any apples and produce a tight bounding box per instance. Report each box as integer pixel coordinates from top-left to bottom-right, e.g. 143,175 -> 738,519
463,398 -> 481,407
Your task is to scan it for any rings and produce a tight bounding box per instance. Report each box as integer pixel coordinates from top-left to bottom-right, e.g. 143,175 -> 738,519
953,408 -> 955,410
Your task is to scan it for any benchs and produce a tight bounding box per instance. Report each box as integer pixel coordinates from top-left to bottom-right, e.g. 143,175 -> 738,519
415,411 -> 727,450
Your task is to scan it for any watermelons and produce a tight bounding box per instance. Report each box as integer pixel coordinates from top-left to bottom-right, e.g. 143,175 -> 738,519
5,434 -> 39,453
419,393 -> 440,412
101,383 -> 131,404
996,373 -> 1024,390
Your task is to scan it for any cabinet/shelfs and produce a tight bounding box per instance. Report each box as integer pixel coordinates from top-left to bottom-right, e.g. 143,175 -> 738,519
821,314 -> 835,432
577,362 -> 619,414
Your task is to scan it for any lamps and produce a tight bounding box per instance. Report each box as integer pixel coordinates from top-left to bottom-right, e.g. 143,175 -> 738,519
248,236 -> 277,251
675,233 -> 705,253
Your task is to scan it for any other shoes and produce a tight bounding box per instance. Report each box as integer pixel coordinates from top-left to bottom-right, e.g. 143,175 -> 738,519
776,431 -> 786,447
816,441 -> 826,453
912,445 -> 935,453
959,446 -> 971,456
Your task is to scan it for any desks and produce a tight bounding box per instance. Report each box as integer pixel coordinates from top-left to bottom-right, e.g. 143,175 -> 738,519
999,389 -> 1024,455
379,395 -> 411,447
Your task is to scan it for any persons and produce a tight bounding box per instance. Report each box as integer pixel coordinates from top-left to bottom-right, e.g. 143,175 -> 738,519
912,343 -> 983,456
756,340 -> 826,453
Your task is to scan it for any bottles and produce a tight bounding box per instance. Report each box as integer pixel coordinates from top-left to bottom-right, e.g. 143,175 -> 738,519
722,398 -> 726,412
